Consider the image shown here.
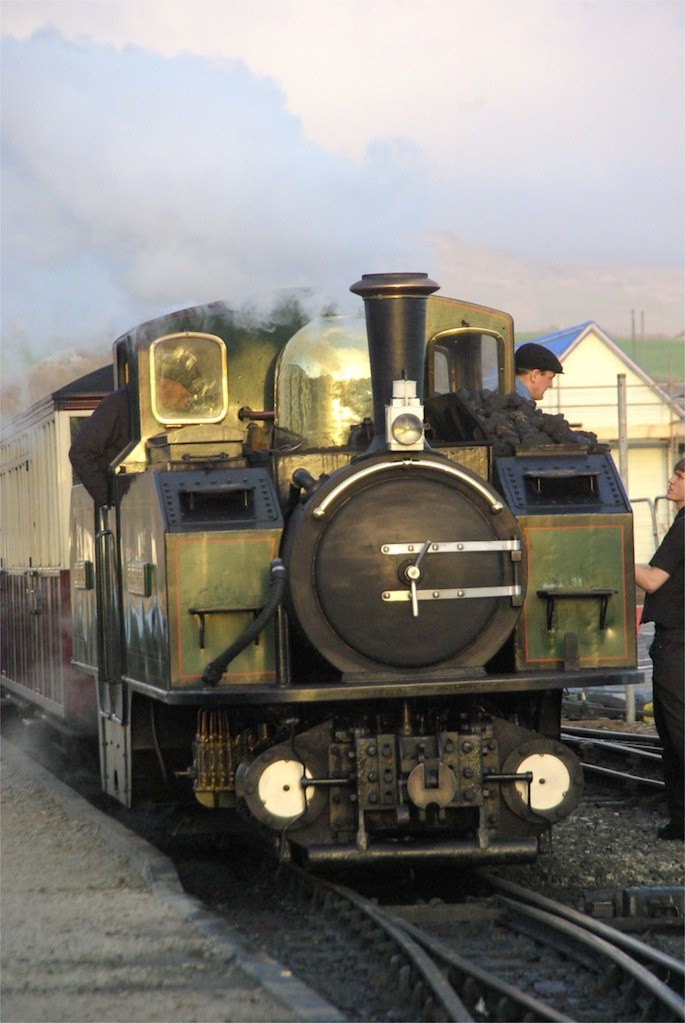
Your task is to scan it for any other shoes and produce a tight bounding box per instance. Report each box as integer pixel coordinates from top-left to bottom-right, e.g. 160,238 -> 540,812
656,820 -> 685,841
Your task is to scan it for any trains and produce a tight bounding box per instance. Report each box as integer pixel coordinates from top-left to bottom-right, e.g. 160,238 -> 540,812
1,266 -> 645,862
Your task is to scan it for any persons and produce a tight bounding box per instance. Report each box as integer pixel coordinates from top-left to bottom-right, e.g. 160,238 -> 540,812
68,354 -> 205,512
482,344 -> 564,400
634,458 -> 685,841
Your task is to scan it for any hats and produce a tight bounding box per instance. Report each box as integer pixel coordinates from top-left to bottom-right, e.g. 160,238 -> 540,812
513,343 -> 564,374
